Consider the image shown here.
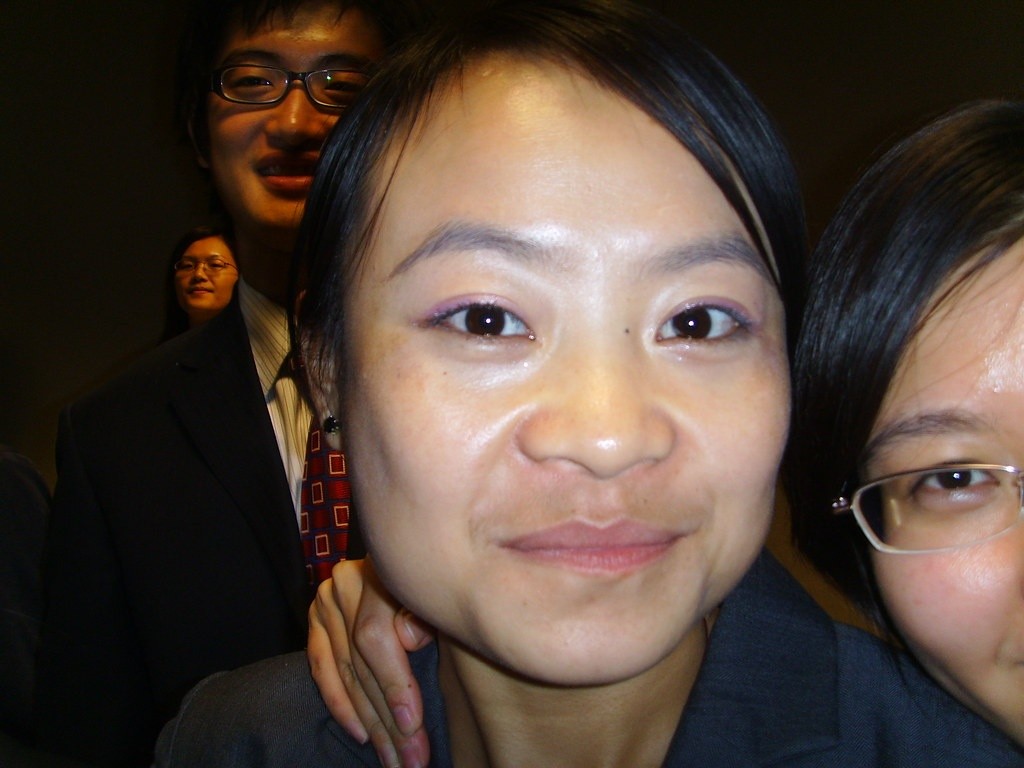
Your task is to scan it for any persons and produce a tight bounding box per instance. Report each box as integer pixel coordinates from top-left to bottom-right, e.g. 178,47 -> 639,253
162,225 -> 242,342
39,1 -> 1024,768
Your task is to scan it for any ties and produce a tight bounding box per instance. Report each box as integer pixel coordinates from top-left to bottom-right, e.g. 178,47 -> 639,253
280,348 -> 352,592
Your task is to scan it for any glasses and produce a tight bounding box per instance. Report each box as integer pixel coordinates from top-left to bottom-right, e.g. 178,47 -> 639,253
173,256 -> 239,273
832,464 -> 1024,556
195,64 -> 374,109
